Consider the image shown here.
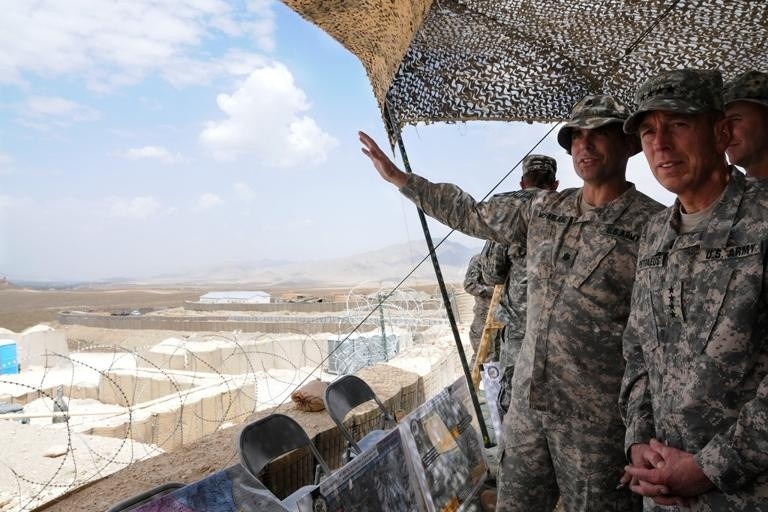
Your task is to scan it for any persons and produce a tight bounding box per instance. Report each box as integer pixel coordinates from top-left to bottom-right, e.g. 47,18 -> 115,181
463,239 -> 501,363
618,68 -> 768,512
723,69 -> 768,177
359,94 -> 668,512
481,154 -> 559,424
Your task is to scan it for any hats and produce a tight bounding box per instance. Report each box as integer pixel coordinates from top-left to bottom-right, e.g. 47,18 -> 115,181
719,70 -> 768,109
557,94 -> 643,157
621,68 -> 725,136
522,154 -> 557,180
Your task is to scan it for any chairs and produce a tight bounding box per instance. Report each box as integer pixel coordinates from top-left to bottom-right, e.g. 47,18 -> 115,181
239,413 -> 334,510
323,375 -> 400,466
